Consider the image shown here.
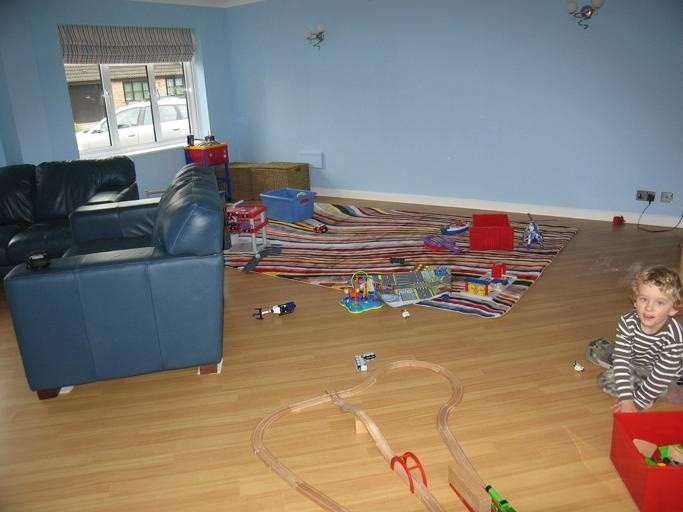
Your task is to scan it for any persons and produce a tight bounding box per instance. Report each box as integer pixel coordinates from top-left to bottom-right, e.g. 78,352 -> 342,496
586,264 -> 682,411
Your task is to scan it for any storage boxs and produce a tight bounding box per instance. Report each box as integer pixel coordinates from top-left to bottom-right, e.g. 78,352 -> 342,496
249,160 -> 310,205
256,186 -> 316,224
213,161 -> 264,202
606,402 -> 682,511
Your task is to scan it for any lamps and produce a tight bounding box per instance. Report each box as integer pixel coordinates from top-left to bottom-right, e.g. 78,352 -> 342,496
562,1 -> 607,30
301,21 -> 331,50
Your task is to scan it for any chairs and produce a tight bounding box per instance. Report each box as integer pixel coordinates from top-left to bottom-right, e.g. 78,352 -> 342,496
0,161 -> 228,400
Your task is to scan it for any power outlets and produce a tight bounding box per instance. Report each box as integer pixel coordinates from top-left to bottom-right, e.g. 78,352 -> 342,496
634,189 -> 657,202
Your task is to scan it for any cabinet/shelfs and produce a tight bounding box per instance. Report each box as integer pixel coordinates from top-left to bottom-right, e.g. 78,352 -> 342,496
183,142 -> 233,204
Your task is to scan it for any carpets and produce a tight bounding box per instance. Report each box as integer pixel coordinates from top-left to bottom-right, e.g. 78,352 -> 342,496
219,198 -> 583,323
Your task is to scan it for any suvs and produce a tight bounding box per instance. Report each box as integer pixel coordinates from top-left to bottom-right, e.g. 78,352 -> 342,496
73,95 -> 190,162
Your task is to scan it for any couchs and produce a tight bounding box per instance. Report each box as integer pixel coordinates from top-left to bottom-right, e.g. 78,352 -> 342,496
0,154 -> 142,285
